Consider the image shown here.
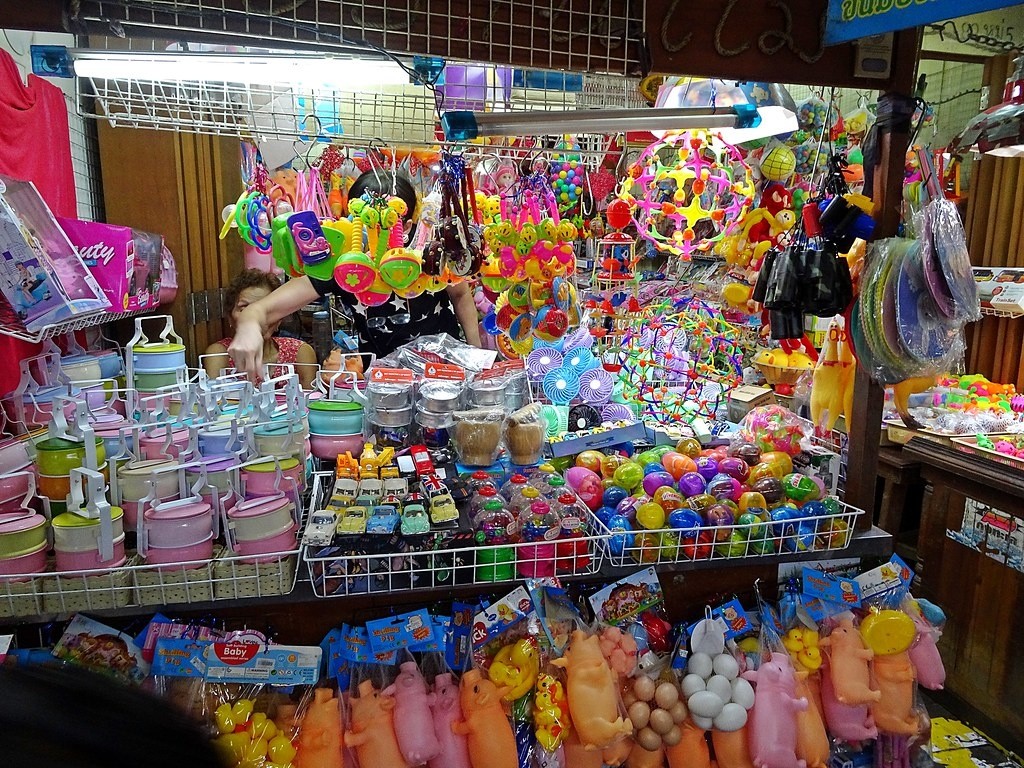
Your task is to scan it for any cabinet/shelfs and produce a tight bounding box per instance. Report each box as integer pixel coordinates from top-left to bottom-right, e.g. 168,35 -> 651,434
86,33 -> 246,385
900,433 -> 1024,766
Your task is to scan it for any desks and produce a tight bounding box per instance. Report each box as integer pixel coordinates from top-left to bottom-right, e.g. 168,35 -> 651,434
876,447 -> 923,537
1,526 -> 895,650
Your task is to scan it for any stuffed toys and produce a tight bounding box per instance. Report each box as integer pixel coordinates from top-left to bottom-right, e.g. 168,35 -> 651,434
734,184 -> 797,285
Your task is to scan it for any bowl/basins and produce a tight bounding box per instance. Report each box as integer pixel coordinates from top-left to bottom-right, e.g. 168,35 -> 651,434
0,314 -> 550,615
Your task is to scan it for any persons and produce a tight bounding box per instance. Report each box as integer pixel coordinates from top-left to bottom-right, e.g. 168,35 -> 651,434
201,267 -> 318,394
225,167 -> 484,378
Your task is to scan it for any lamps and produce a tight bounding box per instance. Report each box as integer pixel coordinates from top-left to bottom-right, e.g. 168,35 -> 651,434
440,102 -> 761,168
61,52 -> 431,119
649,77 -> 799,148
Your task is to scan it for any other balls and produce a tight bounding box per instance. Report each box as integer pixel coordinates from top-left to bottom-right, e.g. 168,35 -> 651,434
759,146 -> 796,181
912,107 -> 933,128
791,182 -> 820,218
800,101 -> 827,126
550,142 -> 584,204
796,145 -> 829,174
